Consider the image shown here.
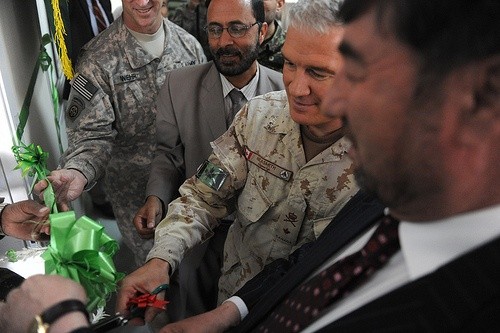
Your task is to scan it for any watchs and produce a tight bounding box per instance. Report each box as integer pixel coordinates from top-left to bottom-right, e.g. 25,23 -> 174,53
29,299 -> 90,333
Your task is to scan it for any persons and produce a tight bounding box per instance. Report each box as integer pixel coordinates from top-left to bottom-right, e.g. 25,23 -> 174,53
0,0 -> 500,333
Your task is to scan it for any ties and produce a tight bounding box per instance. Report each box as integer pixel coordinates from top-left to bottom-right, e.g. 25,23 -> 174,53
247,212 -> 400,333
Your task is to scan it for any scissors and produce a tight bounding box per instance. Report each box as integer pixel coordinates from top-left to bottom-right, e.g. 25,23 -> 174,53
91,285 -> 172,333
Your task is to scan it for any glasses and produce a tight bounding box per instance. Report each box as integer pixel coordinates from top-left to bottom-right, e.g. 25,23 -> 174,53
202,21 -> 263,40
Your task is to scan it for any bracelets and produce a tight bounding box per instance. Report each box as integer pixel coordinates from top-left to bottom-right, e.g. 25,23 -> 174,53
0,203 -> 10,236
